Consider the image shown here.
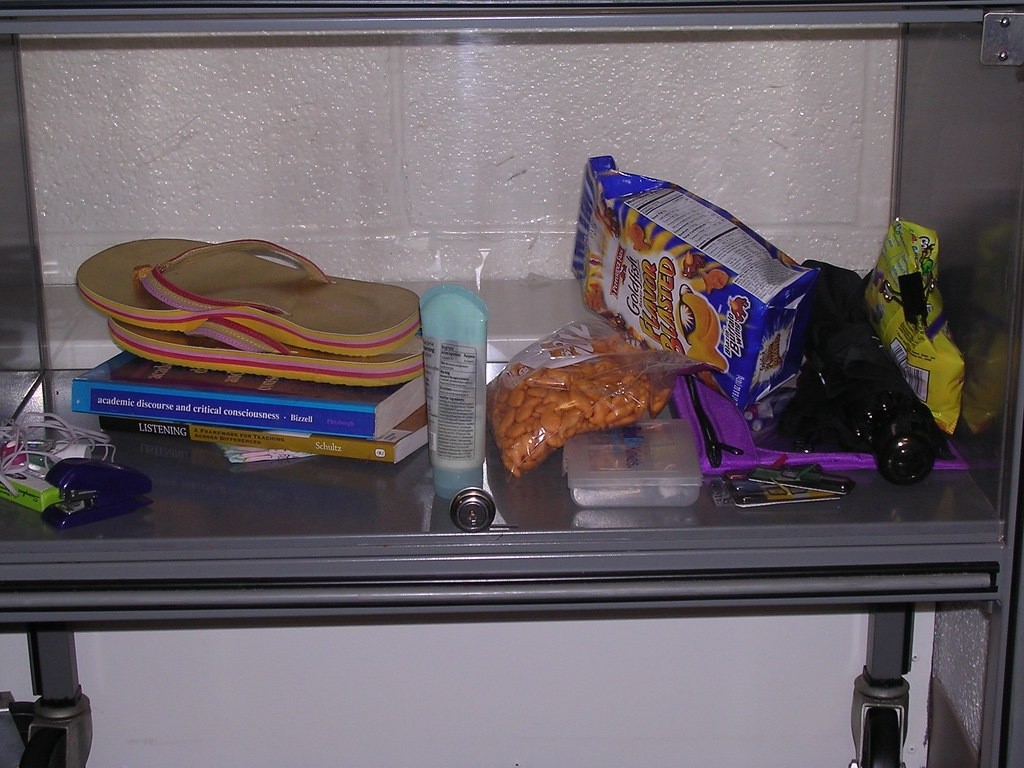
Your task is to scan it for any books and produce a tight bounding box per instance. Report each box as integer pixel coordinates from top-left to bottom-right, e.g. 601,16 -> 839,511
71,349 -> 429,464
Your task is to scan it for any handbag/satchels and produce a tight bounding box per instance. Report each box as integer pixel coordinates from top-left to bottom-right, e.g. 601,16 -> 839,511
669,374 -> 969,476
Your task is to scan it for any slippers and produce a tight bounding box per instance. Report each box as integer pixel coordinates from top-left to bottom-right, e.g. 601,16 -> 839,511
75,238 -> 424,386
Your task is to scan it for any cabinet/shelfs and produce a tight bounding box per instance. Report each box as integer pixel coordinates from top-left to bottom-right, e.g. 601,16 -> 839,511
1,1 -> 1024,768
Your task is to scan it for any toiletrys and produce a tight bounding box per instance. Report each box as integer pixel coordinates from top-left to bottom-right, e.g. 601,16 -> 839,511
421,284 -> 488,499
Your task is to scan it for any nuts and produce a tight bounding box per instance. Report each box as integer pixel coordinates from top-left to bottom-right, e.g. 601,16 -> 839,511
484,333 -> 670,478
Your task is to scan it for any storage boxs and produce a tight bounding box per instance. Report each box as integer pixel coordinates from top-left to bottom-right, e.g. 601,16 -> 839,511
0,462 -> 64,512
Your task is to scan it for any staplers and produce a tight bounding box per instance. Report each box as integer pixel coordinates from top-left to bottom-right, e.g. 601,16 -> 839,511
40,457 -> 154,531
